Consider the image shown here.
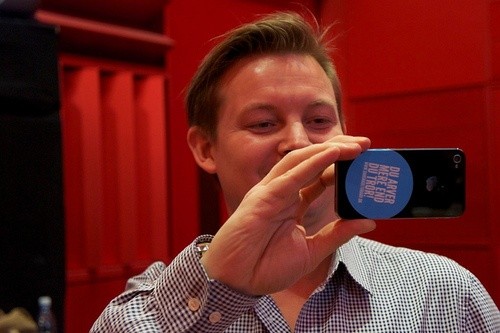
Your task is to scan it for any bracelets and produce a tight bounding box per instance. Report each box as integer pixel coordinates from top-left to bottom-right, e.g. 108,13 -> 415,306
195,240 -> 212,257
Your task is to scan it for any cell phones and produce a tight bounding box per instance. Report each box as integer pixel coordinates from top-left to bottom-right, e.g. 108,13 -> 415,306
335,147 -> 466,218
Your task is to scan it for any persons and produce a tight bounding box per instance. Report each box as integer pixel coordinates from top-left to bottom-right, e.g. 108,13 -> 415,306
88,11 -> 499,332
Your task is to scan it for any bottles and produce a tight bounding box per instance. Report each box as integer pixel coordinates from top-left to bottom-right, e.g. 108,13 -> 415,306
38,295 -> 58,333
